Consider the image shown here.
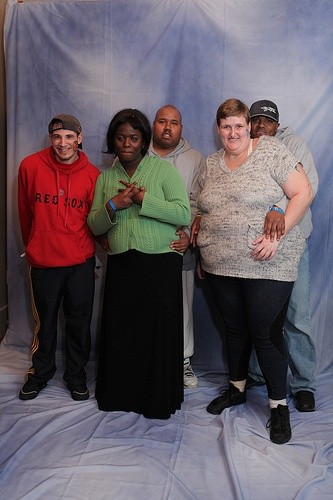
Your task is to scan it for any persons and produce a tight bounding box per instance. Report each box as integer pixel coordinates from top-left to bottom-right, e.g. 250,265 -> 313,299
17,114 -> 103,401
109,199 -> 117,212
87,108 -> 190,419
196,98 -> 313,444
190,100 -> 319,411
96,104 -> 205,388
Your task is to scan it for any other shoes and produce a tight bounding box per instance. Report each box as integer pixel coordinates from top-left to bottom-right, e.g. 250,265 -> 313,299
19,380 -> 47,400
184,364 -> 199,386
294,391 -> 315,412
245,378 -> 266,389
64,370 -> 90,401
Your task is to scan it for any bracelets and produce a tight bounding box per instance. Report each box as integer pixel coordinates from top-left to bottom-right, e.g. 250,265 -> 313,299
270,206 -> 285,215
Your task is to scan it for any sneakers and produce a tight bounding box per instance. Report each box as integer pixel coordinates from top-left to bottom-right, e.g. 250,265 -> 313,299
206,382 -> 247,415
266,404 -> 291,445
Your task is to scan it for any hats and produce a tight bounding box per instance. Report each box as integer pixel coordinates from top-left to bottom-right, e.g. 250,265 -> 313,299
250,100 -> 279,122
48,114 -> 83,149
